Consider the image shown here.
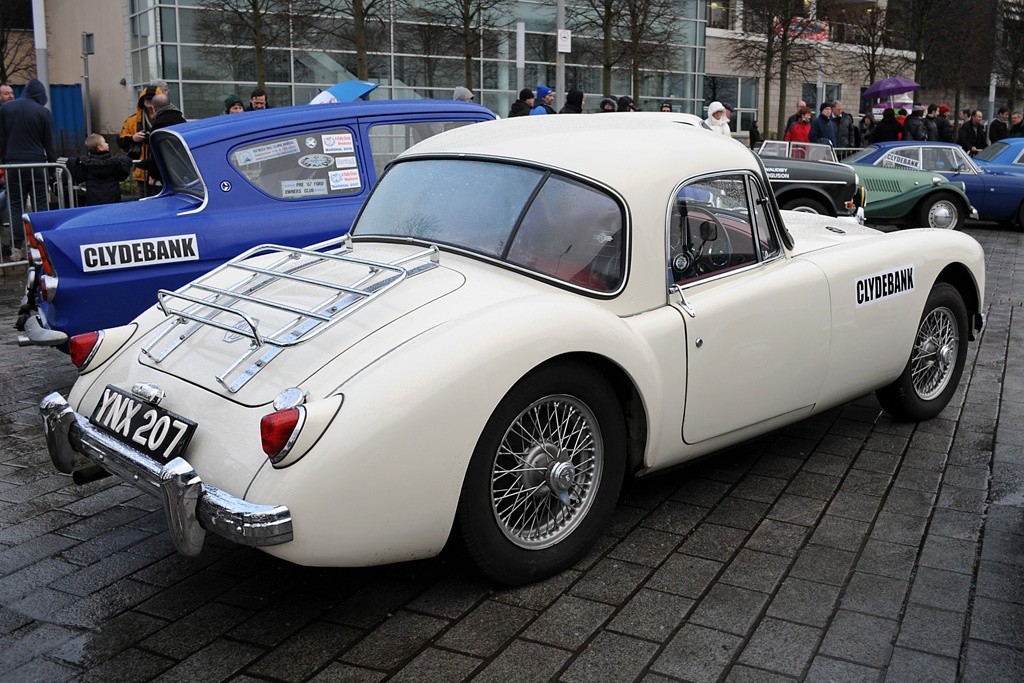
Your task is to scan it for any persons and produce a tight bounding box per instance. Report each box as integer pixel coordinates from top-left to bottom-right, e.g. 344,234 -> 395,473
116,85 -> 170,199
508,88 -> 534,118
557,87 -> 585,115
1,77 -> 60,262
243,88 -> 274,111
597,86 -> 1023,160
148,78 -> 170,99
452,85 -> 474,102
75,134 -> 134,206
528,85 -> 556,116
133,94 -> 189,186
0,82 -> 17,227
219,93 -> 244,116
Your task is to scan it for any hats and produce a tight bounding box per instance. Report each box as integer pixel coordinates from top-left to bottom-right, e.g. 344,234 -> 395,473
520,88 -> 534,101
661,103 -> 672,112
914,105 -> 924,117
537,86 -> 552,99
940,104 -> 951,113
820,102 -> 832,114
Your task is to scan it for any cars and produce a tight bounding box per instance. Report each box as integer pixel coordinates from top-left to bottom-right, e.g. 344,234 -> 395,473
756,141 -> 980,232
749,153 -> 867,226
25,95 -> 512,352
39,110 -> 988,587
932,136 -> 1024,168
839,141 -> 1024,234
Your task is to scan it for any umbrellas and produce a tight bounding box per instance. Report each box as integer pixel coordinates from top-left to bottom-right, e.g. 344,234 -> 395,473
862,74 -> 921,111
308,80 -> 382,106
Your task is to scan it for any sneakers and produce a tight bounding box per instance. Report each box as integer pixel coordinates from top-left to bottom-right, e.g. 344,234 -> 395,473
10,246 -> 25,261
2,218 -> 10,226
